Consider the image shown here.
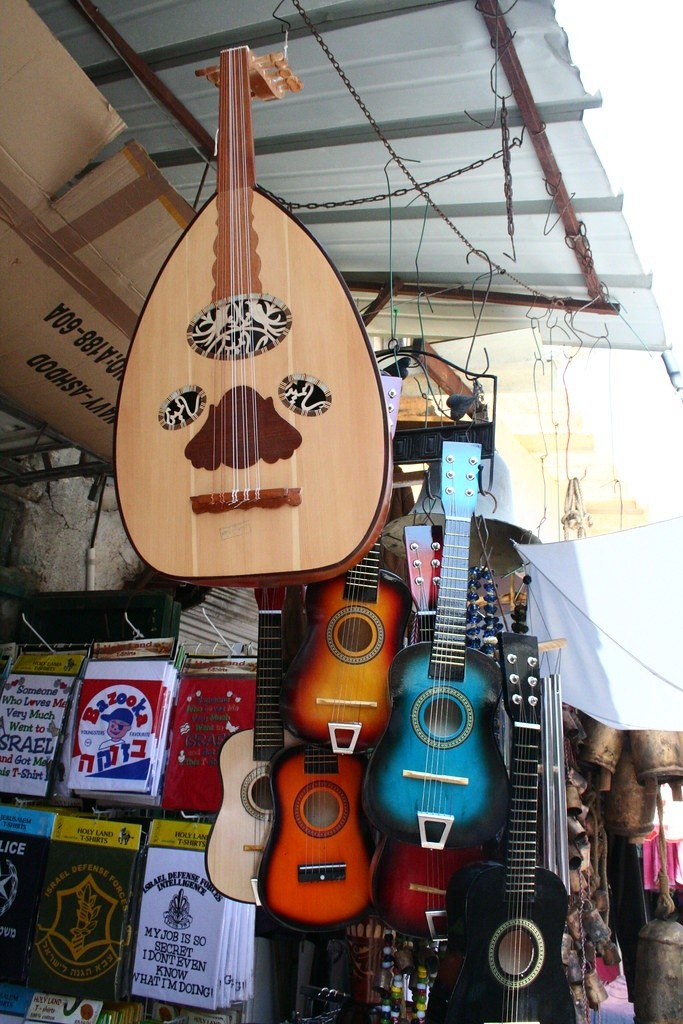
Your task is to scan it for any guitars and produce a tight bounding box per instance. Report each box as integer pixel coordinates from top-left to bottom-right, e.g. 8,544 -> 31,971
428,636 -> 576,1024
113,43 -> 395,585
202,596 -> 294,906
287,529 -> 392,743
362,830 -> 447,936
257,734 -> 369,930
365,440 -> 517,847
